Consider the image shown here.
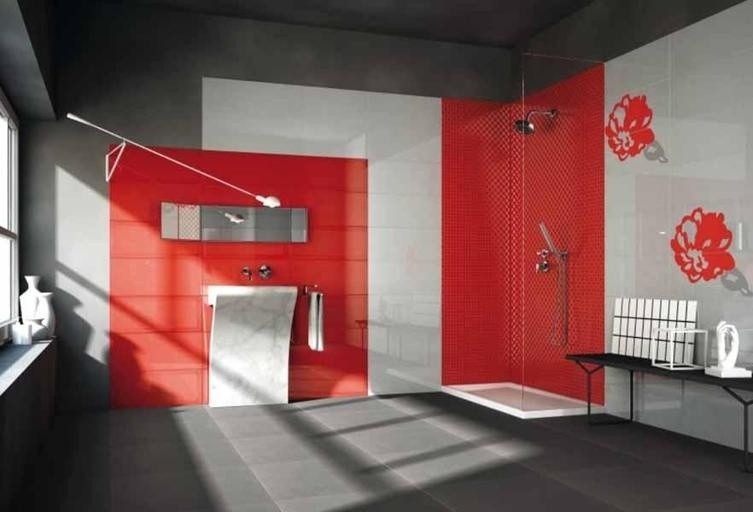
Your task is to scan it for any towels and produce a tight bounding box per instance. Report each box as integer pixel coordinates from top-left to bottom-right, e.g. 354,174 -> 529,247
307,292 -> 326,352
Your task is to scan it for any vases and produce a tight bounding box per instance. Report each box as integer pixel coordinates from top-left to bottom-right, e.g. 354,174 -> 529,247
18,275 -> 43,339
27,318 -> 47,340
36,293 -> 56,338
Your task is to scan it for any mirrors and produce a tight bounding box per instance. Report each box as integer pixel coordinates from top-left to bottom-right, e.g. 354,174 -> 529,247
161,201 -> 310,243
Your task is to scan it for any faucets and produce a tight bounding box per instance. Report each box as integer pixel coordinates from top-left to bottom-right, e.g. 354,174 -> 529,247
258,265 -> 272,279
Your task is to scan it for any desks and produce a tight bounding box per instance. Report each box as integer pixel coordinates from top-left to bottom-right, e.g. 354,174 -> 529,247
565,352 -> 753,472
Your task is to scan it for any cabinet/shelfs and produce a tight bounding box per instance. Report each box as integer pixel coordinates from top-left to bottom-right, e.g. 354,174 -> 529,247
0,332 -> 57,510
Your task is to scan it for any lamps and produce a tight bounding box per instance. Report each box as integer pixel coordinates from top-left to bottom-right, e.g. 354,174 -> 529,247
64,108 -> 282,210
203,207 -> 245,225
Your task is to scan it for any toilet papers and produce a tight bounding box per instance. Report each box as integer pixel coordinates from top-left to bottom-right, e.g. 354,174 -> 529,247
10,321 -> 33,346
